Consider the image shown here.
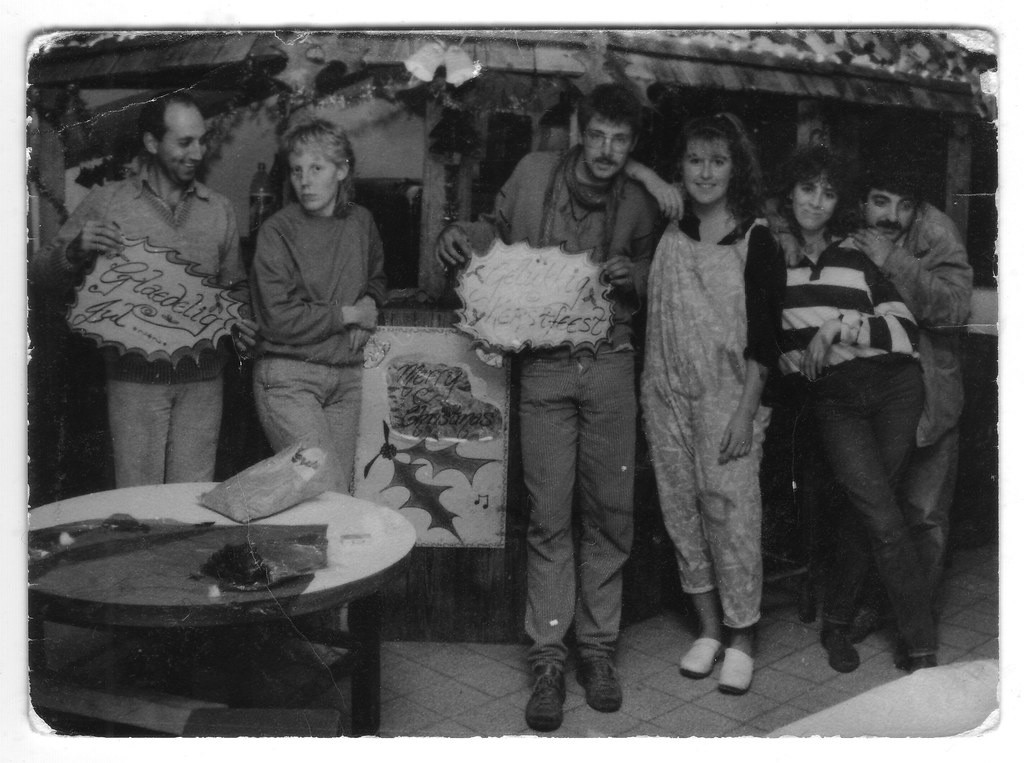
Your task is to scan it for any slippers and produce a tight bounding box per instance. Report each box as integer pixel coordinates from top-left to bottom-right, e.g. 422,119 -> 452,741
678,638 -> 725,679
718,648 -> 755,694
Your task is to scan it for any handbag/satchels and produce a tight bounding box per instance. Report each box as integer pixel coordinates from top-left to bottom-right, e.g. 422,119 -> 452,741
195,441 -> 328,525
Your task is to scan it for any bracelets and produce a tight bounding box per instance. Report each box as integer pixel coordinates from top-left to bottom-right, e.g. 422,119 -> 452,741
629,159 -> 647,176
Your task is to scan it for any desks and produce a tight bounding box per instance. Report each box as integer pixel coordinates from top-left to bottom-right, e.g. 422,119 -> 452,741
29,483 -> 416,736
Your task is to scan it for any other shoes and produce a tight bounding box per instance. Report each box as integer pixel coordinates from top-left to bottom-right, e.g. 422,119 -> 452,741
841,608 -> 884,644
820,626 -> 861,673
893,639 -> 936,674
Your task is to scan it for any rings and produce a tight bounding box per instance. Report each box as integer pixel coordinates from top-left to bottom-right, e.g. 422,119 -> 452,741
740,439 -> 746,446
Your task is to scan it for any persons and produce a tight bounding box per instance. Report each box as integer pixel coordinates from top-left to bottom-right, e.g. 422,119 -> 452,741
26,93 -> 260,495
381,158 -> 497,284
433,81 -> 655,731
245,159 -> 269,201
766,132 -> 973,675
247,118 -> 387,630
626,108 -> 787,695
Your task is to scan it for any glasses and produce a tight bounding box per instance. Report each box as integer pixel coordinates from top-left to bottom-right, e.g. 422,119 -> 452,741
584,129 -> 629,155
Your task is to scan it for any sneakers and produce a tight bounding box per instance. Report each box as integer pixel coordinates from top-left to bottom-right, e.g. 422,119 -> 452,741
524,664 -> 567,732
574,658 -> 622,713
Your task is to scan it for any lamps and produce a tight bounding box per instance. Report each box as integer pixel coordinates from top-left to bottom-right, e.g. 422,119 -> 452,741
402,35 -> 479,88
